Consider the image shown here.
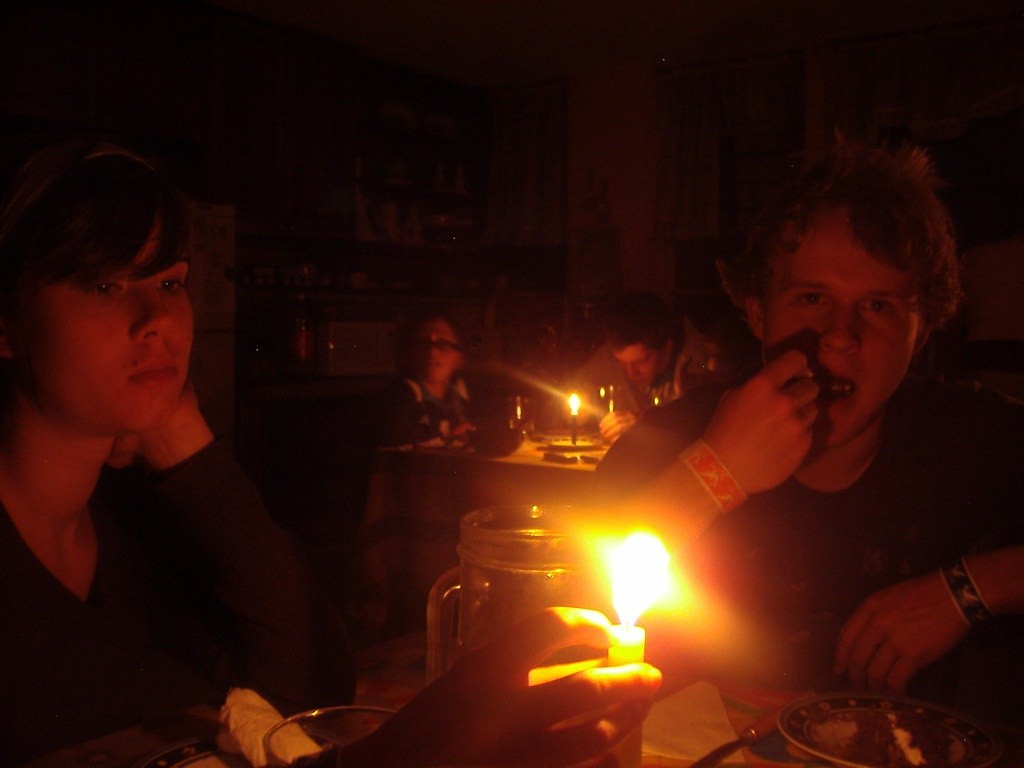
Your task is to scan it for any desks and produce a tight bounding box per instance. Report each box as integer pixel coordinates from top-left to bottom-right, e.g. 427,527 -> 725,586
365,421 -> 611,617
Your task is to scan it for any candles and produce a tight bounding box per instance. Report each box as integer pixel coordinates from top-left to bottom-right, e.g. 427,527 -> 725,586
604,533 -> 672,768
568,393 -> 582,445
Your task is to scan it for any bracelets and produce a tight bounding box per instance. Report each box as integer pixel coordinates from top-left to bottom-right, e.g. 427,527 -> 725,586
678,435 -> 749,517
943,555 -> 997,638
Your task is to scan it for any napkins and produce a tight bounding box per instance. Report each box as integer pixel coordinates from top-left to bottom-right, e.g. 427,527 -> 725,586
215,689 -> 321,768
643,681 -> 744,766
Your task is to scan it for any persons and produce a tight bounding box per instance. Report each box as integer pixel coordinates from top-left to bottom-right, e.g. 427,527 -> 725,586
357,233 -> 760,528
572,135 -> 1024,734
2,144 -> 665,768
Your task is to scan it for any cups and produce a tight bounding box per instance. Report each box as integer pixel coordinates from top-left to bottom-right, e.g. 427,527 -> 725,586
261,704 -> 403,768
423,502 -> 590,691
594,383 -> 627,424
507,395 -> 536,430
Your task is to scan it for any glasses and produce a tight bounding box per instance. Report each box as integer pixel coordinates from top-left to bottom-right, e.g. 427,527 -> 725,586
416,338 -> 461,353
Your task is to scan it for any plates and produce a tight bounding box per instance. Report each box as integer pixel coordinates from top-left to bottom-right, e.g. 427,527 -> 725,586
545,437 -> 603,450
777,691 -> 1002,768
